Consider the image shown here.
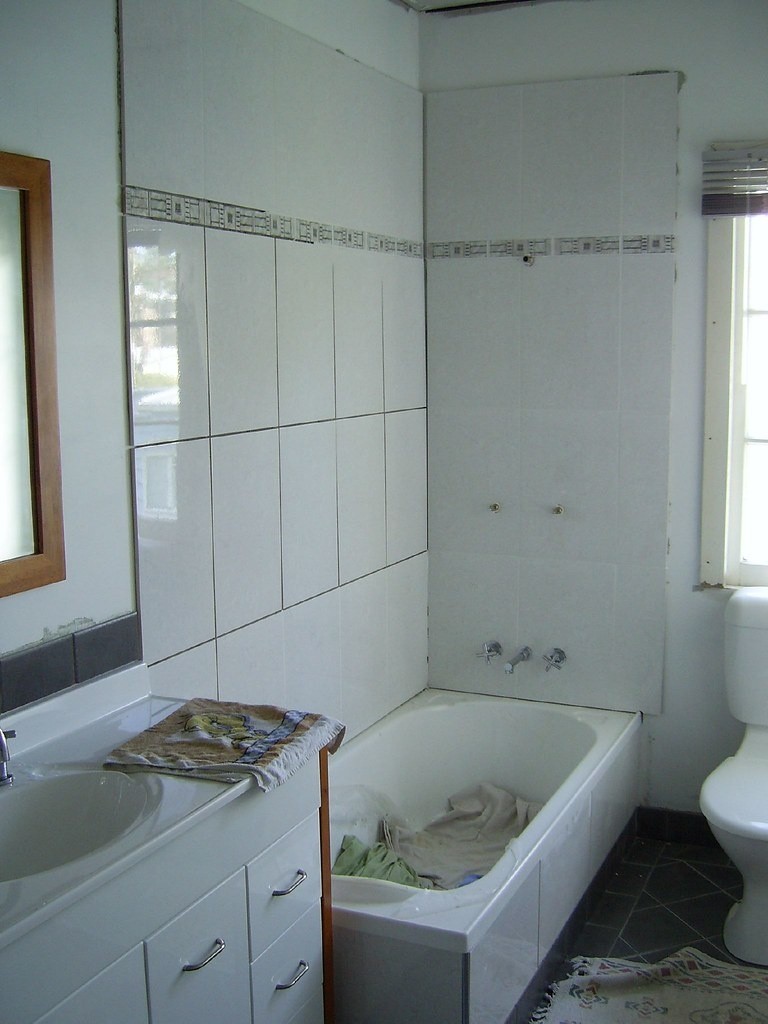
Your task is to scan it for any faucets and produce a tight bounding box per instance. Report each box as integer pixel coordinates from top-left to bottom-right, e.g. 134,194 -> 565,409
0,728 -> 17,787
504,646 -> 533,676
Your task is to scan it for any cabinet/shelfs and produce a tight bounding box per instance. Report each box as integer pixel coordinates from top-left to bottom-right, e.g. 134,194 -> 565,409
0,745 -> 331,1024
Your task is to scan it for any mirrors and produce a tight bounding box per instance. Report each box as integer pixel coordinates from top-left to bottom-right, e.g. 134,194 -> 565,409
0,150 -> 66,596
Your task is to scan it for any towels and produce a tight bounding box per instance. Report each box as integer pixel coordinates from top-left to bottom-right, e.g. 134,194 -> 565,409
101,697 -> 345,794
331,779 -> 546,891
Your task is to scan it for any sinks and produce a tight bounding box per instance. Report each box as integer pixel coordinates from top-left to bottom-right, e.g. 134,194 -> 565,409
0,756 -> 166,918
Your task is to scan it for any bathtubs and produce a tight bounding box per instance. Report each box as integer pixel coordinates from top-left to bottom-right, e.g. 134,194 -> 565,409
326,684 -> 645,1008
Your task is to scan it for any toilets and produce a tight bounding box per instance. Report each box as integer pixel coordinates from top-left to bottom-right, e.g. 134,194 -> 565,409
696,586 -> 768,970
